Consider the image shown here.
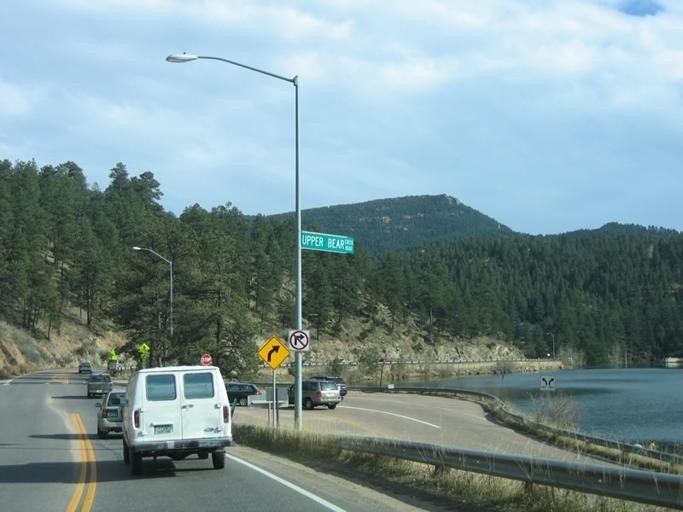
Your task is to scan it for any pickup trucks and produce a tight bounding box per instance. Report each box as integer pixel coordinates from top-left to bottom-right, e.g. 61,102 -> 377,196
86,373 -> 113,396
77,362 -> 93,374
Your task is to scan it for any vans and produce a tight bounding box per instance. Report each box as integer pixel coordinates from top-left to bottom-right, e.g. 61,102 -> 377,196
224,381 -> 261,407
118,363 -> 234,477
288,374 -> 347,411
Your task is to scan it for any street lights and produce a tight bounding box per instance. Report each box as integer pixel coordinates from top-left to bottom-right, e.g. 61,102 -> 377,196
164,50 -> 305,432
131,244 -> 176,340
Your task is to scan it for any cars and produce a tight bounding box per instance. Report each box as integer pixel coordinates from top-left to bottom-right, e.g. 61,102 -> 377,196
94,389 -> 126,440
